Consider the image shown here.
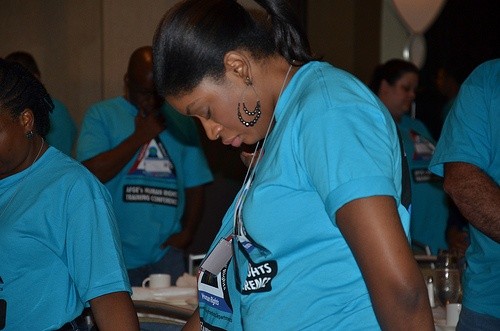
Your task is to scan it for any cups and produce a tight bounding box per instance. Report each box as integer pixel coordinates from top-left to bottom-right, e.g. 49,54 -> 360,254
445,304 -> 463,328
141,272 -> 172,292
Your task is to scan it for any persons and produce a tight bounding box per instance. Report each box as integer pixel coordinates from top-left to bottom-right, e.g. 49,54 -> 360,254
76,44 -> 215,330
427,58 -> 500,330
151,1 -> 436,331
6,52 -> 77,159
0,59 -> 141,331
368,59 -> 450,254
445,206 -> 471,270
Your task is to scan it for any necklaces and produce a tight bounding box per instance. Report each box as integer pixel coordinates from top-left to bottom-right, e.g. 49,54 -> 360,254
32,137 -> 45,164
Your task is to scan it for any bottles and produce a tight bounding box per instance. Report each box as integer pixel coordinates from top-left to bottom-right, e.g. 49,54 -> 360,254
437,247 -> 461,305
426,273 -> 436,309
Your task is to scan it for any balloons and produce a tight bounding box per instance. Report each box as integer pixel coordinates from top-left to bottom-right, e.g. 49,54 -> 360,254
393,0 -> 442,35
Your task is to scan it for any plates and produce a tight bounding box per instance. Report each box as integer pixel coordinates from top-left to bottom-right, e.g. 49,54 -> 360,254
156,288 -> 198,304
